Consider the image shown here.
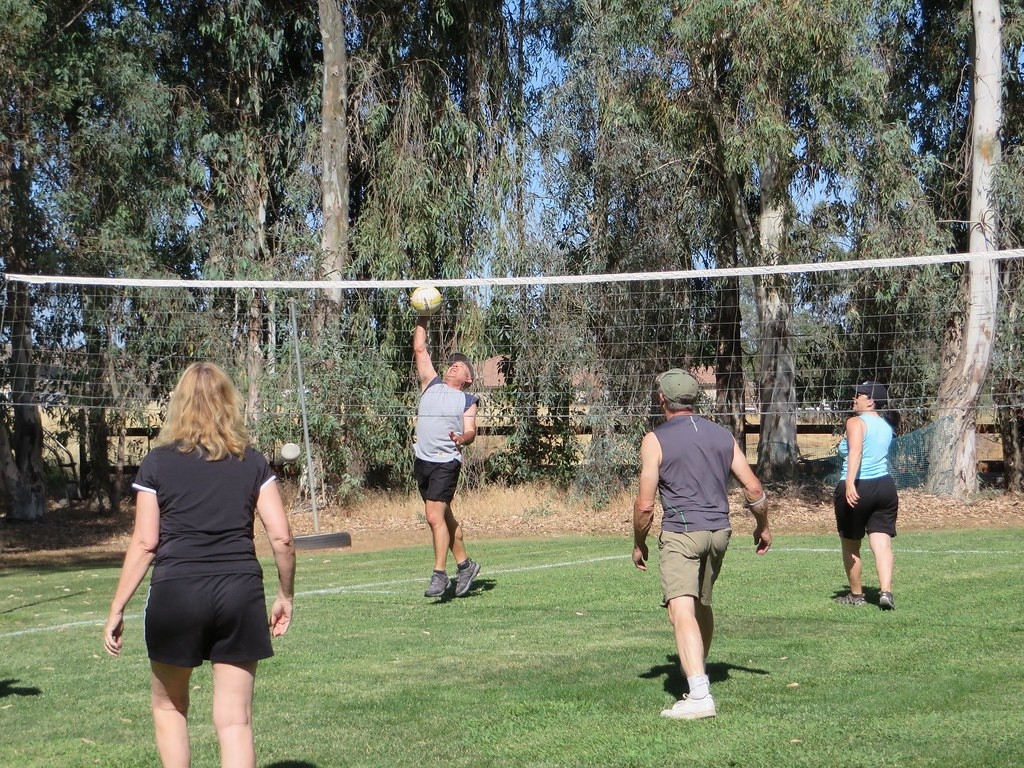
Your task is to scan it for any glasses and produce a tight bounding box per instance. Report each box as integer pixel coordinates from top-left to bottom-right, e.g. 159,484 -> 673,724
856,392 -> 868,398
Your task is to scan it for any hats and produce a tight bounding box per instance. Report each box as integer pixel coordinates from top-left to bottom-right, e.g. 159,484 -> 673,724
448,353 -> 474,388
659,368 -> 699,404
852,381 -> 887,406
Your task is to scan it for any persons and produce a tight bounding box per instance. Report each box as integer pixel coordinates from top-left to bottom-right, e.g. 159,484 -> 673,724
833,381 -> 898,610
414,313 -> 480,597
630,369 -> 773,719
105,361 -> 297,768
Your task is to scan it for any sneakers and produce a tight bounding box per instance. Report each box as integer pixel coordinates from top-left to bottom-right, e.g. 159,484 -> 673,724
879,591 -> 895,610
454,560 -> 481,597
660,693 -> 717,720
834,592 -> 863,606
424,572 -> 452,597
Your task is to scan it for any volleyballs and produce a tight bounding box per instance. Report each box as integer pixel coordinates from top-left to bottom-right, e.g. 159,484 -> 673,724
412,287 -> 442,316
281,442 -> 300,461
